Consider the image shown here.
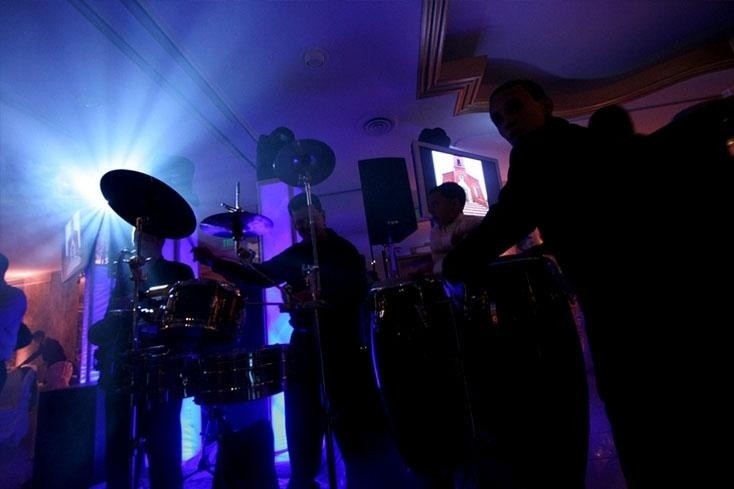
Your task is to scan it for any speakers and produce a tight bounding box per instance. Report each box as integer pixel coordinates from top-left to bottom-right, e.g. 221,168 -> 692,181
31,386 -> 110,489
357,156 -> 419,246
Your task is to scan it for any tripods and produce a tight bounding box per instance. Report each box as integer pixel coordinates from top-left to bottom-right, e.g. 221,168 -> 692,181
183,403 -> 217,480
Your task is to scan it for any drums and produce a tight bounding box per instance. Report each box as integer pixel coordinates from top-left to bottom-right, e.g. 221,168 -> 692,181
160,277 -> 242,349
373,281 -> 428,340
182,345 -> 286,407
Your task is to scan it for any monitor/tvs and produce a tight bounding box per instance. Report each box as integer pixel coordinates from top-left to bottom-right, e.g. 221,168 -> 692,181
411,140 -> 503,219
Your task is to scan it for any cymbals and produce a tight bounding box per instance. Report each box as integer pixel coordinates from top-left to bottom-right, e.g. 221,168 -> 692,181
200,211 -> 274,243
100,169 -> 197,240
274,139 -> 335,187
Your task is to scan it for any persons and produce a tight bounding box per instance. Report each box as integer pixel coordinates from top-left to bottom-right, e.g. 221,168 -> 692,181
106,231 -> 195,489
0,253 -> 73,489
191,192 -> 367,489
